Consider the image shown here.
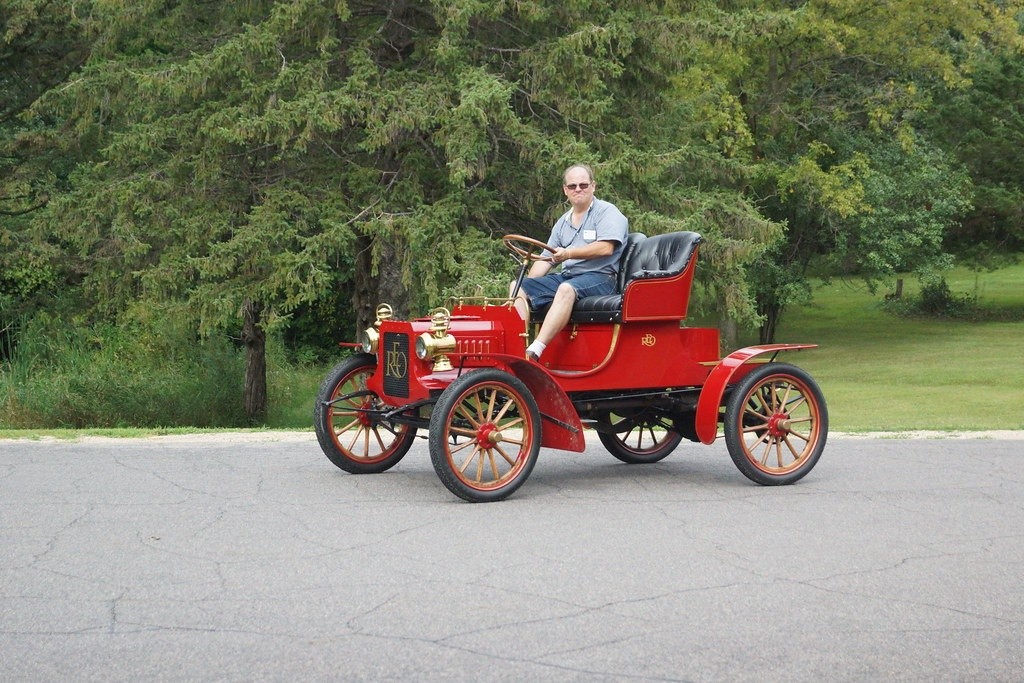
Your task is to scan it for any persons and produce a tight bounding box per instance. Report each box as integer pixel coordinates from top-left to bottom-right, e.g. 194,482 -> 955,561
509,162 -> 629,362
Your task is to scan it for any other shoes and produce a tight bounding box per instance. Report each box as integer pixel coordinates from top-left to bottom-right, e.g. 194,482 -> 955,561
526,351 -> 539,362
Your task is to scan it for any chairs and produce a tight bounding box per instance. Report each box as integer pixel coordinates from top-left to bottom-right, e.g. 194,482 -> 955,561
530,231 -> 701,323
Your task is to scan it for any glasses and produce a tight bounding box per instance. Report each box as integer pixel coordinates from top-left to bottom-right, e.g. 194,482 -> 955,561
567,183 -> 591,189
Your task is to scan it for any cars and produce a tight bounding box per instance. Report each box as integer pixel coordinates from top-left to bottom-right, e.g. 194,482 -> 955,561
313,233 -> 828,502
